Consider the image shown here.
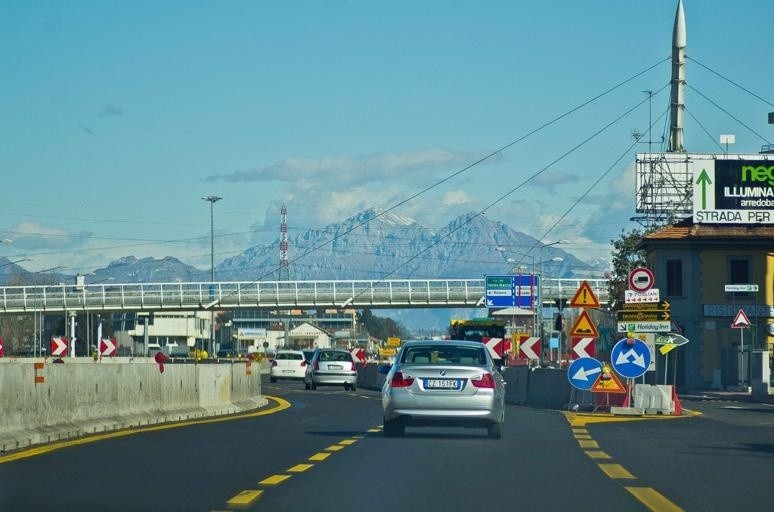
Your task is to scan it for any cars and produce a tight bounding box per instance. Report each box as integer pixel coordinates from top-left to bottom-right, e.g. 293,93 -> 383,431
304,347 -> 358,393
376,339 -> 509,440
302,349 -> 317,364
268,349 -> 308,383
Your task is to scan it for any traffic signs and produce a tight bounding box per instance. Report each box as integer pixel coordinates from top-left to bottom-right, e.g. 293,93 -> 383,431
616,320 -> 672,333
484,274 -> 514,308
654,331 -> 690,347
691,159 -> 774,224
513,273 -> 540,309
617,309 -> 671,322
618,299 -> 672,311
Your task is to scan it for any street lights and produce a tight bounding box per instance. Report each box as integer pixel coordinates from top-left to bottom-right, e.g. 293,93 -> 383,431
0,237 -> 116,357
201,194 -> 225,358
